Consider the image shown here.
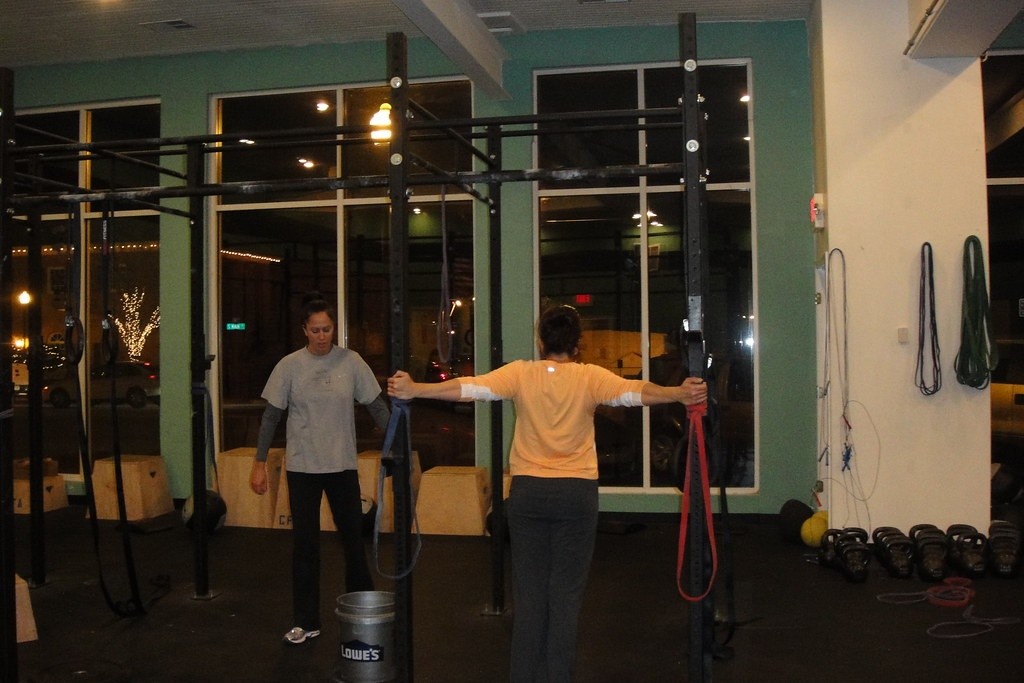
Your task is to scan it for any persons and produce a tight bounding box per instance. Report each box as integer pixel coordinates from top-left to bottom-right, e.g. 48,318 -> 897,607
386,307 -> 707,683
250,301 -> 394,644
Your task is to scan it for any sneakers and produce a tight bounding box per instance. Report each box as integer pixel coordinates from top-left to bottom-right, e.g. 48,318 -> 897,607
282,627 -> 320,644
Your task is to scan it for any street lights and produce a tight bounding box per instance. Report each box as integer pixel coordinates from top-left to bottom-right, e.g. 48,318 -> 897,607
19,288 -> 30,354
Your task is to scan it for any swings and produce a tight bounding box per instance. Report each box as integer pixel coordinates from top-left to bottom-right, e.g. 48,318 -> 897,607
116,235 -> 194,537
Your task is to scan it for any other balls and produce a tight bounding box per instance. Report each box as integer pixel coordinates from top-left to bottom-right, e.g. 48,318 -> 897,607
781,500 -> 828,547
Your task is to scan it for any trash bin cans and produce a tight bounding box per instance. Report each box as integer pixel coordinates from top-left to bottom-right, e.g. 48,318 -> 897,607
333,591 -> 396,683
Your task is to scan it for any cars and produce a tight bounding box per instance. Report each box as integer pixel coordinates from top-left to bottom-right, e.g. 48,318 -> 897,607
359,350 -> 388,390
424,347 -> 476,409
627,348 -> 755,446
990,337 -> 1024,446
41,360 -> 162,410
